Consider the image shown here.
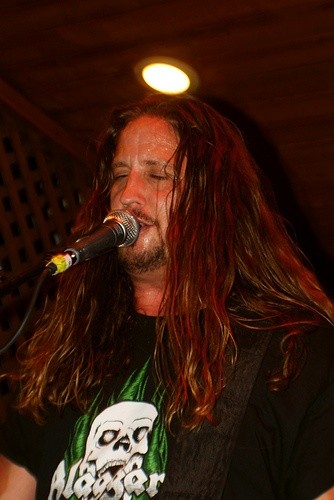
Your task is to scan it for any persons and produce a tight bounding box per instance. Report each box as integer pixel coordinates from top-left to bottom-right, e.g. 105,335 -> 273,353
0,92 -> 334,500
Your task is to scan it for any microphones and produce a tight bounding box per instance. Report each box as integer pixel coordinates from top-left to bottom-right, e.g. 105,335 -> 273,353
45,208 -> 139,275
